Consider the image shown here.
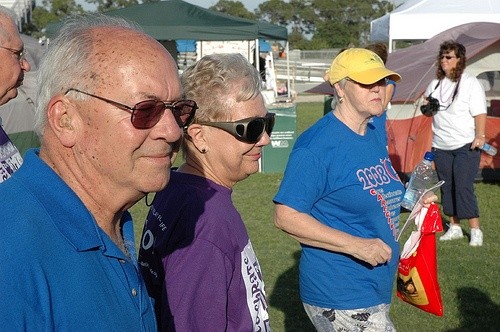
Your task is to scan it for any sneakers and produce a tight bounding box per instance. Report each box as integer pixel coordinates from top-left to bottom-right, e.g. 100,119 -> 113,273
469,228 -> 483,247
439,222 -> 464,241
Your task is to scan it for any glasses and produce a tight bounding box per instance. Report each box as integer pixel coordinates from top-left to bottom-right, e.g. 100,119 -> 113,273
440,56 -> 457,60
194,113 -> 275,144
0,45 -> 23,60
64,87 -> 199,130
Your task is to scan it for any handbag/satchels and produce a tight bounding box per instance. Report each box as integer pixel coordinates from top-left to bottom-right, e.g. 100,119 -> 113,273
396,203 -> 445,317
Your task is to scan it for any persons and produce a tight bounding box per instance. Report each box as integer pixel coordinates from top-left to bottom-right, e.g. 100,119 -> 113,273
267,60 -> 274,89
0,13 -> 198,332
273,48 -> 438,332
364,43 -> 396,152
137,54 -> 276,332
419,39 -> 487,246
0,6 -> 31,184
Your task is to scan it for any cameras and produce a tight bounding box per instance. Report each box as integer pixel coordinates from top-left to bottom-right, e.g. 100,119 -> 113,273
420,96 -> 440,117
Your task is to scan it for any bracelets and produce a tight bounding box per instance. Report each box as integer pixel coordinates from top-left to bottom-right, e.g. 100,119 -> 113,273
476,134 -> 486,138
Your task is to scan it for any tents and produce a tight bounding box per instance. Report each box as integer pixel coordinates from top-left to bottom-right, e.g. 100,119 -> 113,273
46,0 -> 291,173
370,0 -> 500,53
383,22 -> 500,173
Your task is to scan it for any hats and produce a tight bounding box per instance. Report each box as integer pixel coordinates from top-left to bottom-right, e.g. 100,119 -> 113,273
329,48 -> 402,85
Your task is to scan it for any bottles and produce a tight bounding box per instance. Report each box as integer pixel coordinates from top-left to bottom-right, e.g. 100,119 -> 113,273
479,143 -> 497,156
400,151 -> 434,211
